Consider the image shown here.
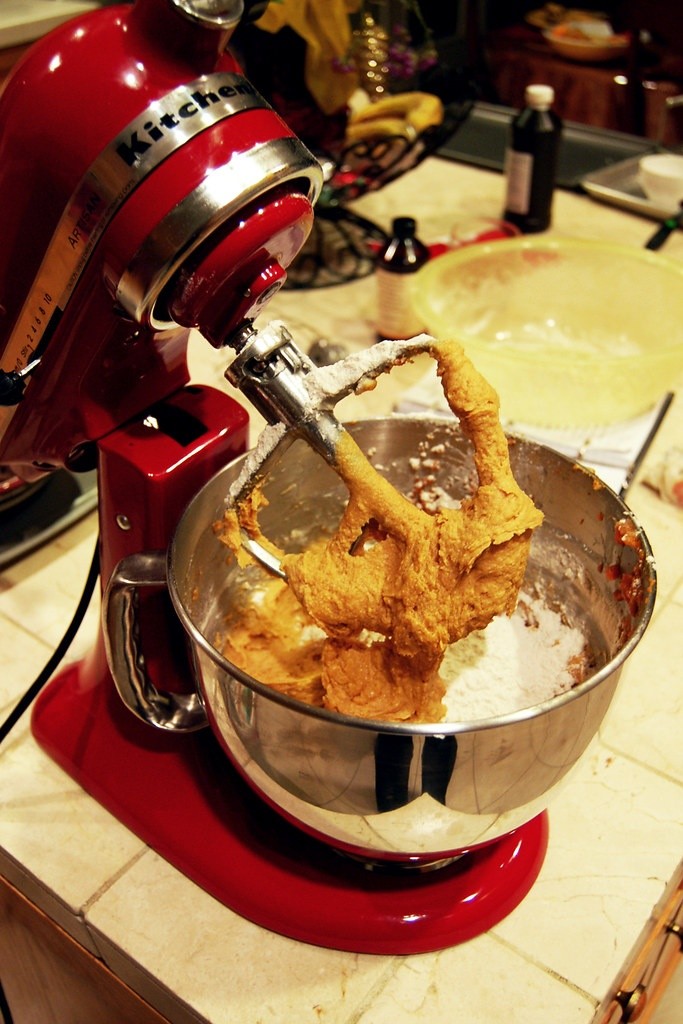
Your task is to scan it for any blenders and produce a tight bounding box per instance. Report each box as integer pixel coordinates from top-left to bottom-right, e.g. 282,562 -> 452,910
0,0 -> 551,960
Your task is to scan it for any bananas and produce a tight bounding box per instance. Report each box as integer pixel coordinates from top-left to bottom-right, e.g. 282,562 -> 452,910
346,90 -> 445,145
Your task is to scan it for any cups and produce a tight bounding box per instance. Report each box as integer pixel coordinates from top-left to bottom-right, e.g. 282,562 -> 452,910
638,153 -> 683,209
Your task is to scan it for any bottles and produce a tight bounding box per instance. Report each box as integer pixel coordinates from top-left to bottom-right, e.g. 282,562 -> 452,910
377,217 -> 429,342
504,82 -> 565,234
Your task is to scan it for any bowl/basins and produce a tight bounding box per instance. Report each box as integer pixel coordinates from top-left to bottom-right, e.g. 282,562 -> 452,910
98,413 -> 658,862
543,29 -> 631,62
407,234 -> 683,426
